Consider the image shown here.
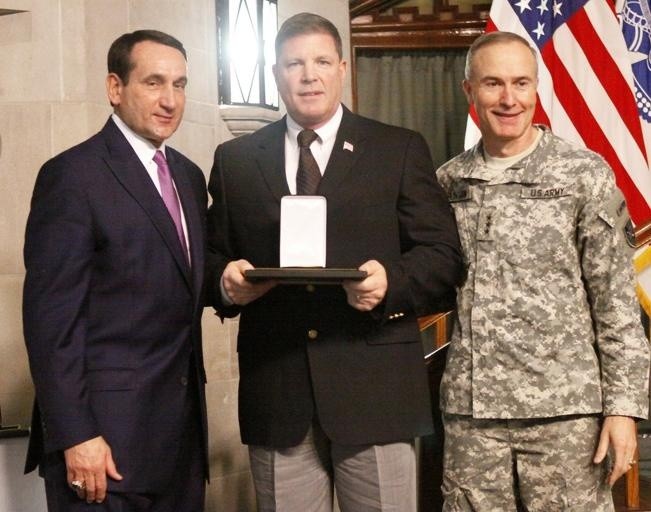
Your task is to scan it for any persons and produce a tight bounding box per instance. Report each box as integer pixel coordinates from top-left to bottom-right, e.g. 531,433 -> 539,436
23,30 -> 210,512
436,31 -> 651,511
208,14 -> 468,512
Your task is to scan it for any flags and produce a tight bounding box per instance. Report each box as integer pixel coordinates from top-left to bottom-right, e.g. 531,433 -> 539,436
464,0 -> 651,321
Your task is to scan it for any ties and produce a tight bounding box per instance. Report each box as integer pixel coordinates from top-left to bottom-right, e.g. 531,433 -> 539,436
297,130 -> 322,197
152,150 -> 190,268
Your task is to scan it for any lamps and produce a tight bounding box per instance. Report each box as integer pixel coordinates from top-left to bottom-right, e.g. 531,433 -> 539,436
216,0 -> 280,112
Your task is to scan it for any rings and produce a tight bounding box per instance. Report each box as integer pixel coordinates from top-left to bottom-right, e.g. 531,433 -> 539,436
356,296 -> 359,302
71,480 -> 85,490
